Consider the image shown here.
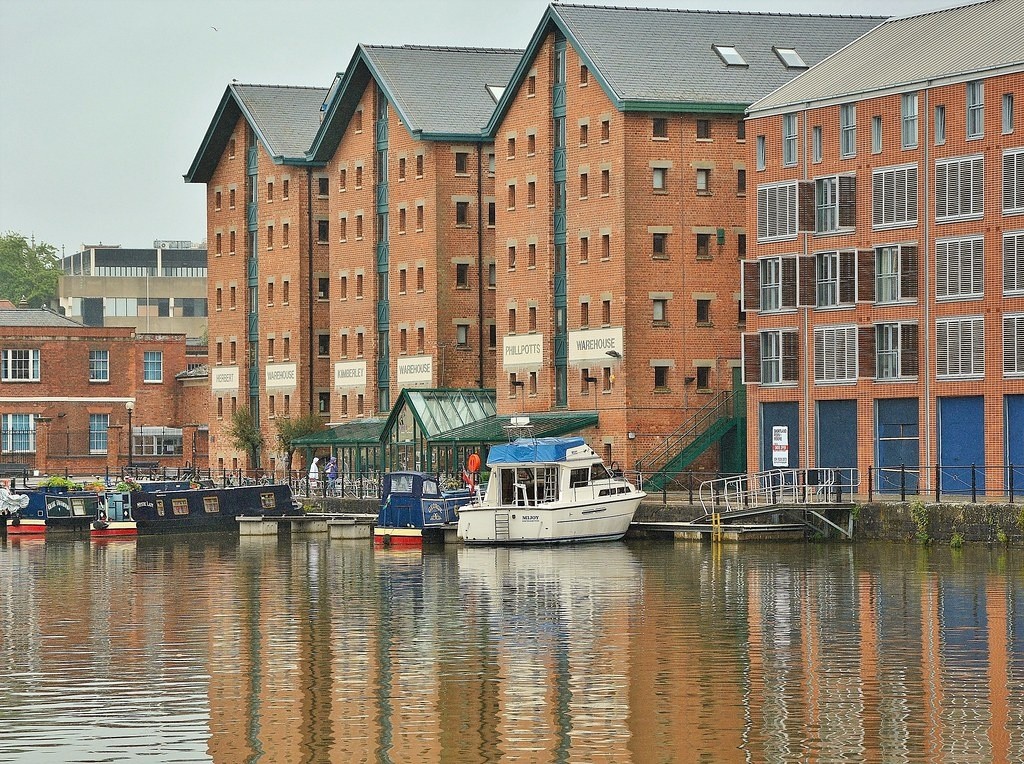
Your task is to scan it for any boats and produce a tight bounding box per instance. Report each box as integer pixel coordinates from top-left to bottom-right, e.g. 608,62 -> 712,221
456,425 -> 647,545
375,470 -> 488,545
113,477 -> 217,492
88,485 -> 308,538
0,477 -> 100,533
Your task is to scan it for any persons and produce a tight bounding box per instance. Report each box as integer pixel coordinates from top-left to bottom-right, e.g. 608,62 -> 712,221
608,461 -> 623,477
340,458 -> 349,478
190,475 -> 206,489
308,457 -> 319,496
324,458 -> 338,496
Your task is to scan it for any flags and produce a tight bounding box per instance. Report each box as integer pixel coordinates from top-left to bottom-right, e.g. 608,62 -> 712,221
463,467 -> 475,494
383,494 -> 391,510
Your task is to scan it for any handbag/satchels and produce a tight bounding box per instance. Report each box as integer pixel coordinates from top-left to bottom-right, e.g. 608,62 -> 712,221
326,462 -> 331,473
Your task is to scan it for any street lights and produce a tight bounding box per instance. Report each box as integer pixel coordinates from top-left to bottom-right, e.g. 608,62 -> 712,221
125,400 -> 135,476
